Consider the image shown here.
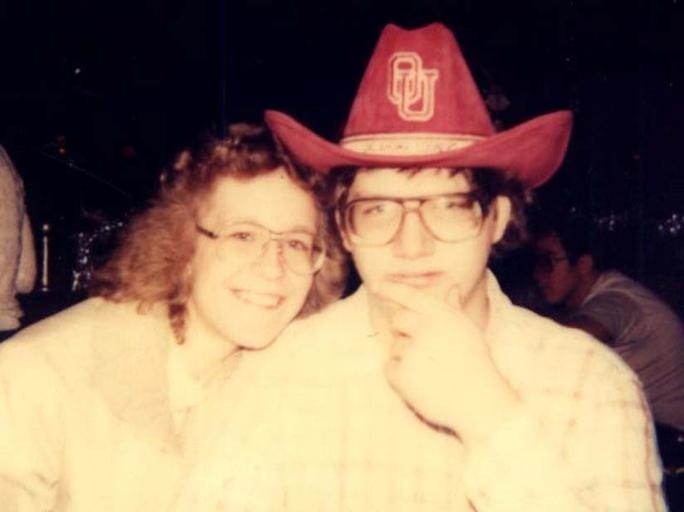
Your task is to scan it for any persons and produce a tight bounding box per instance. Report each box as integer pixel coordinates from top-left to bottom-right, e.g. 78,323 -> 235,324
0,146 -> 36,344
213,18 -> 670,510
527,214 -> 682,495
0,113 -> 350,510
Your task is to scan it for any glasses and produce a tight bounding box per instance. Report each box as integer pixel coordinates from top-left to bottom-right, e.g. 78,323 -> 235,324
339,189 -> 485,247
195,221 -> 326,275
530,255 -> 567,271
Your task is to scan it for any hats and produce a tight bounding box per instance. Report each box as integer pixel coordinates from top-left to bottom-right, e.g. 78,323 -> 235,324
264,23 -> 575,189
